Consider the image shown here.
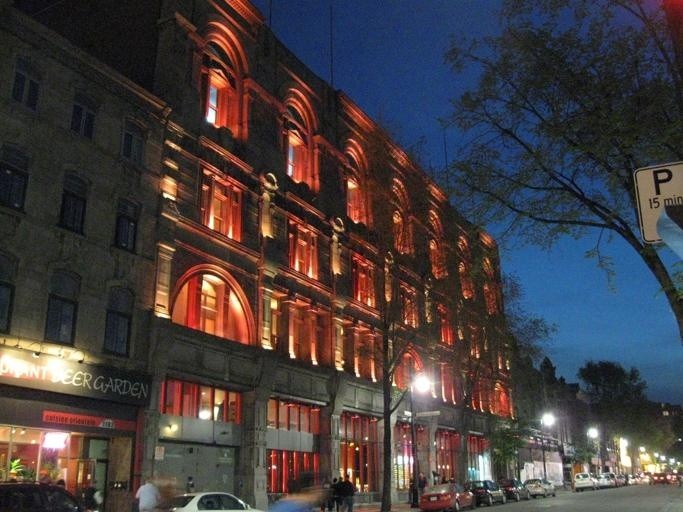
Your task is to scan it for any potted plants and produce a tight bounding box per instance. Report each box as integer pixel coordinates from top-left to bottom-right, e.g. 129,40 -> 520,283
7,458 -> 22,479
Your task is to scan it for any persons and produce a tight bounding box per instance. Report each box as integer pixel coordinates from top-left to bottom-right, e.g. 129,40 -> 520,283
0,476 -> 65,489
408,471 -> 456,508
134,476 -> 161,512
81,479 -> 104,512
319,474 -> 354,512
186,476 -> 196,493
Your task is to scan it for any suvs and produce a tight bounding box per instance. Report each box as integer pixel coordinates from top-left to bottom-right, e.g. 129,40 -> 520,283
469,480 -> 507,507
499,479 -> 530,503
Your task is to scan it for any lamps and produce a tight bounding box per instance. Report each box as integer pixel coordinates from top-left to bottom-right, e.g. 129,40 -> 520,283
26,341 -> 46,361
70,350 -> 90,363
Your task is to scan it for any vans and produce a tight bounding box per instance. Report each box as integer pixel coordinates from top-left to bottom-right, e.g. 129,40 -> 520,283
573,473 -> 599,491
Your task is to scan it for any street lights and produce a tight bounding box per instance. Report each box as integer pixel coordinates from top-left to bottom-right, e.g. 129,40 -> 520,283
584,425 -> 680,474
407,354 -> 432,508
538,412 -> 554,479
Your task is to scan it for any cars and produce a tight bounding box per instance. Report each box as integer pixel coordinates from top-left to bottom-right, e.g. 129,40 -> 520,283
0,480 -> 84,512
150,491 -> 264,512
417,484 -> 476,511
598,471 -> 680,488
522,478 -> 555,499
